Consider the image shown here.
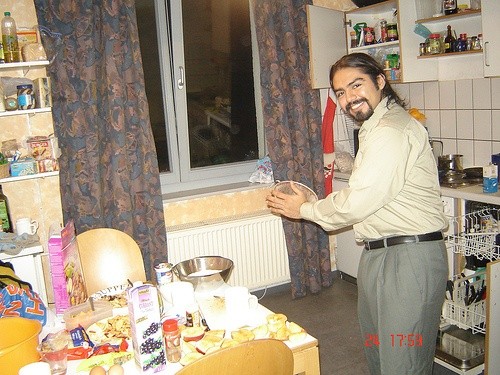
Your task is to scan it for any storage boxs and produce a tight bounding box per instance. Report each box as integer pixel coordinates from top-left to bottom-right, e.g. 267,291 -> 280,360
483,165 -> 498,193
48,219 -> 86,317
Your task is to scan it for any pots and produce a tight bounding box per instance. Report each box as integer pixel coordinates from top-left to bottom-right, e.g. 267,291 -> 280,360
438,154 -> 483,187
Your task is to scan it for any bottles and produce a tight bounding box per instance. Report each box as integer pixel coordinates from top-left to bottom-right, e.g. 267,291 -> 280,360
1,12 -> 20,64
419,24 -> 483,56
163,319 -> 182,363
383,61 -> 391,80
0,184 -> 14,233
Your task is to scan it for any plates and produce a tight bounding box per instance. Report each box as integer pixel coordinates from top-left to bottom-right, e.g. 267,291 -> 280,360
86,282 -> 135,347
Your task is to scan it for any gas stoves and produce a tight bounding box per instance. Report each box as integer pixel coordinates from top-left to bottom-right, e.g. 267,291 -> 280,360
439,180 -> 482,189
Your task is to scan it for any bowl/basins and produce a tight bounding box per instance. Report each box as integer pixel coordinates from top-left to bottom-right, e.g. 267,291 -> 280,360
173,256 -> 234,292
0,317 -> 42,375
62,299 -> 113,334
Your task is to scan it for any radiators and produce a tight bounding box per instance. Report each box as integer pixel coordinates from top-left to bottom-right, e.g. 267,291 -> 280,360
164,209 -> 292,293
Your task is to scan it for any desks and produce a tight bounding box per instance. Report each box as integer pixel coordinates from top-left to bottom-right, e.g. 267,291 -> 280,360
39,281 -> 322,375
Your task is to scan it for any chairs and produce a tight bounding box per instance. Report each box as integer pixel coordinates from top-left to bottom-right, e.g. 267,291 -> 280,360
76,227 -> 147,298
177,339 -> 295,374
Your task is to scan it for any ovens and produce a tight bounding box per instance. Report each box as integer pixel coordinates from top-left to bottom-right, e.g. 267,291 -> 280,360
440,196 -> 458,280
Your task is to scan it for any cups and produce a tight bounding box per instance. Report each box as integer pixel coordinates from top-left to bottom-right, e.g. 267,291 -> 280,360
415,0 -> 481,20
19,361 -> 52,375
185,305 -> 200,328
37,338 -> 69,375
153,262 -> 174,284
15,217 -> 39,235
223,286 -> 259,318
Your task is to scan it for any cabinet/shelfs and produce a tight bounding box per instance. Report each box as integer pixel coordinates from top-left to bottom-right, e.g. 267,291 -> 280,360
0,60 -> 60,182
307,0 -> 500,89
0,243 -> 47,308
331,177 -> 500,374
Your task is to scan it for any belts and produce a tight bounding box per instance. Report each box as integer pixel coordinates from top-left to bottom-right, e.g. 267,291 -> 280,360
365,231 -> 443,250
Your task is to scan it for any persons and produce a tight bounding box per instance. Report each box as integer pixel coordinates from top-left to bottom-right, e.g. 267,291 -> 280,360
266,53 -> 449,375
